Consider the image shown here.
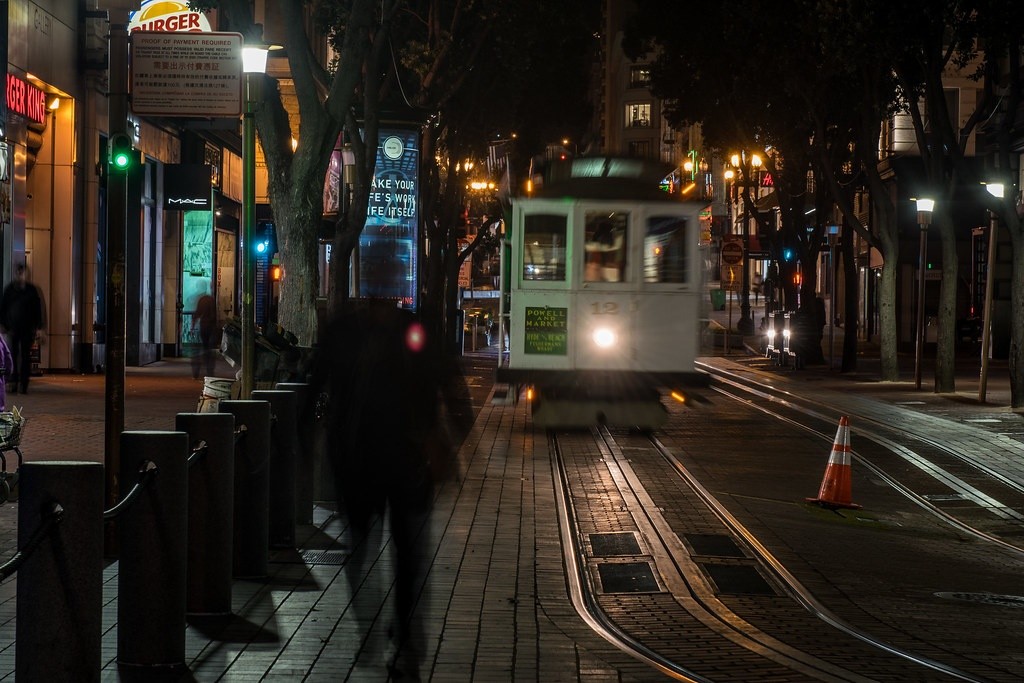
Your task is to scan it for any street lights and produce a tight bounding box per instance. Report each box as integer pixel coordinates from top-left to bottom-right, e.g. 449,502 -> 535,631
236,21 -> 284,400
723,151 -> 765,335
977,178 -> 1005,406
909,196 -> 936,391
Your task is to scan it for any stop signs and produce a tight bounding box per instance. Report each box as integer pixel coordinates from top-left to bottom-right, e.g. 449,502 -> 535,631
720,238 -> 745,266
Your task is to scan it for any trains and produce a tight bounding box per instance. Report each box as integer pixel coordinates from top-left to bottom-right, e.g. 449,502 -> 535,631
496,153 -> 706,380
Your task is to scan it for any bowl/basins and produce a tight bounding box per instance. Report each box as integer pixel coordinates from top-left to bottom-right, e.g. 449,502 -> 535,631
203,376 -> 237,401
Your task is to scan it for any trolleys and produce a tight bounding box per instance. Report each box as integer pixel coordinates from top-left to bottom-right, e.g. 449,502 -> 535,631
0,404 -> 29,507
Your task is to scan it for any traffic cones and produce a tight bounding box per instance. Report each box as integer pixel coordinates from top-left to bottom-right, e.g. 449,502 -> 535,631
806,415 -> 862,509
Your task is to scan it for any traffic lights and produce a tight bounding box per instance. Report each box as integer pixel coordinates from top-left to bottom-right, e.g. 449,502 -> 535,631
255,221 -> 267,255
783,249 -> 794,261
269,265 -> 280,282
758,170 -> 780,188
792,273 -> 800,284
109,132 -> 133,172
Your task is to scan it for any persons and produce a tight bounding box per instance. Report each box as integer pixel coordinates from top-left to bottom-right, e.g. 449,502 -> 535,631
186,280 -> 232,382
0,264 -> 47,395
311,236 -> 482,683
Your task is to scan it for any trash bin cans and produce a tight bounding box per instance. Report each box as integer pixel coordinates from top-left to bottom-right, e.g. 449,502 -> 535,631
710,289 -> 726,311
